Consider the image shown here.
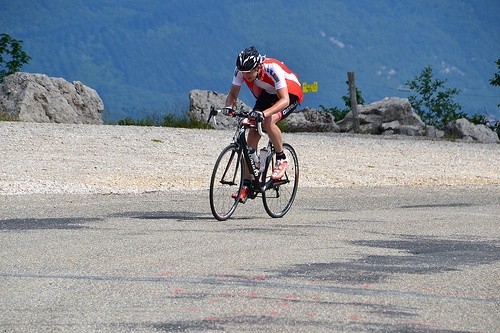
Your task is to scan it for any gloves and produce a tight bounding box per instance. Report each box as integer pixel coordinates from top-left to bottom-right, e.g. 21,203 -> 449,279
248,110 -> 266,123
222,106 -> 235,118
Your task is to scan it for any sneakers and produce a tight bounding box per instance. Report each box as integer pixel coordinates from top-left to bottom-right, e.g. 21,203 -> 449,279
232,188 -> 249,199
272,158 -> 288,180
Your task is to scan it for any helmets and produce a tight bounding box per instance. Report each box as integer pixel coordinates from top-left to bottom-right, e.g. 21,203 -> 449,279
236,45 -> 262,72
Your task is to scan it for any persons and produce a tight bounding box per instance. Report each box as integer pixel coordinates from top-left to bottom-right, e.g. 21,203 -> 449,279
223,47 -> 303,204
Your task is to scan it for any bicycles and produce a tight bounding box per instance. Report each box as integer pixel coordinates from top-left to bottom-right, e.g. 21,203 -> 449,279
202,105 -> 300,222
